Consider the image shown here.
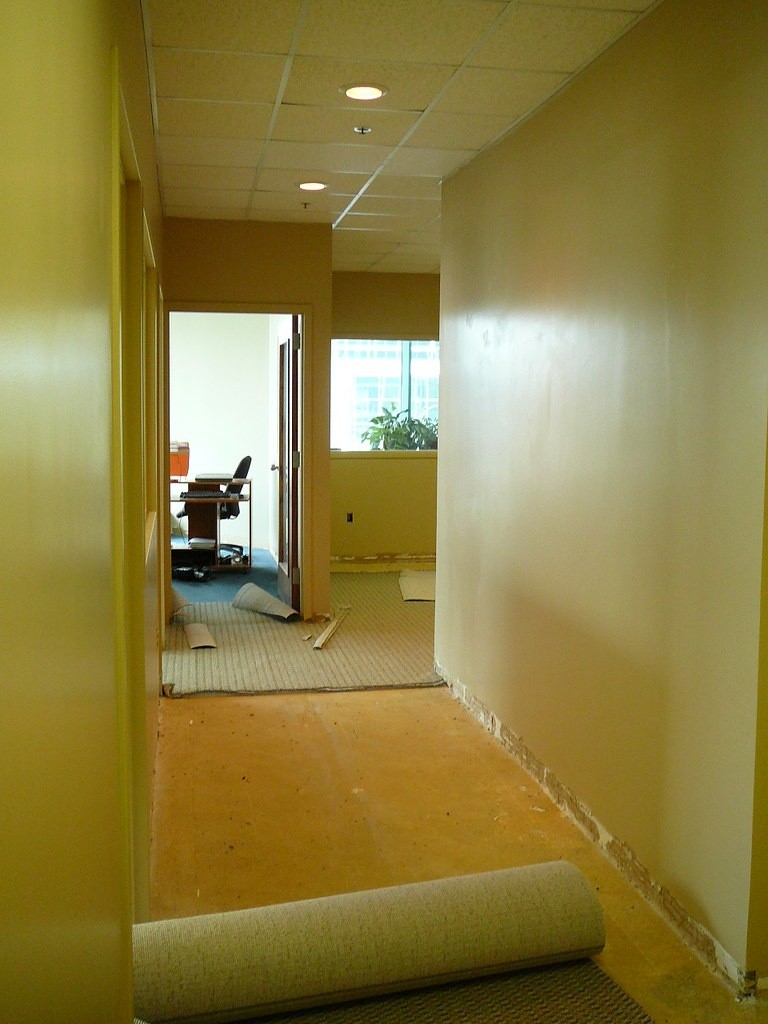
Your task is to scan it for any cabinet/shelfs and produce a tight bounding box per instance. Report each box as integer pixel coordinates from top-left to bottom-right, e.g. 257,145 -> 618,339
172,479 -> 251,573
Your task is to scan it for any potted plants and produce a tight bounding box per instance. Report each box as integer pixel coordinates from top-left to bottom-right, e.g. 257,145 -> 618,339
361,401 -> 438,451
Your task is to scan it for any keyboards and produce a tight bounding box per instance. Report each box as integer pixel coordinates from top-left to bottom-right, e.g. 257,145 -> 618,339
180,490 -> 231,498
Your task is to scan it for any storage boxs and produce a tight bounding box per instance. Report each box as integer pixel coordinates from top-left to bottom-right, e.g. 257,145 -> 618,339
170,440 -> 190,477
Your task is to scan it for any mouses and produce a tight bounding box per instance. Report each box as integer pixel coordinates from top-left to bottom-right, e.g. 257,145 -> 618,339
231,493 -> 239,499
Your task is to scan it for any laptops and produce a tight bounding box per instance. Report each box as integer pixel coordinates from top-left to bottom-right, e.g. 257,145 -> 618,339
195,473 -> 233,482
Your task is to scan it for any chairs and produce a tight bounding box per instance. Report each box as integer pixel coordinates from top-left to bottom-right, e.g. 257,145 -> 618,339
178,456 -> 252,567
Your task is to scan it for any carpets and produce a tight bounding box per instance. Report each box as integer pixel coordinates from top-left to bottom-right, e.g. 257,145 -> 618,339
131,859 -> 660,1024
161,572 -> 449,700
